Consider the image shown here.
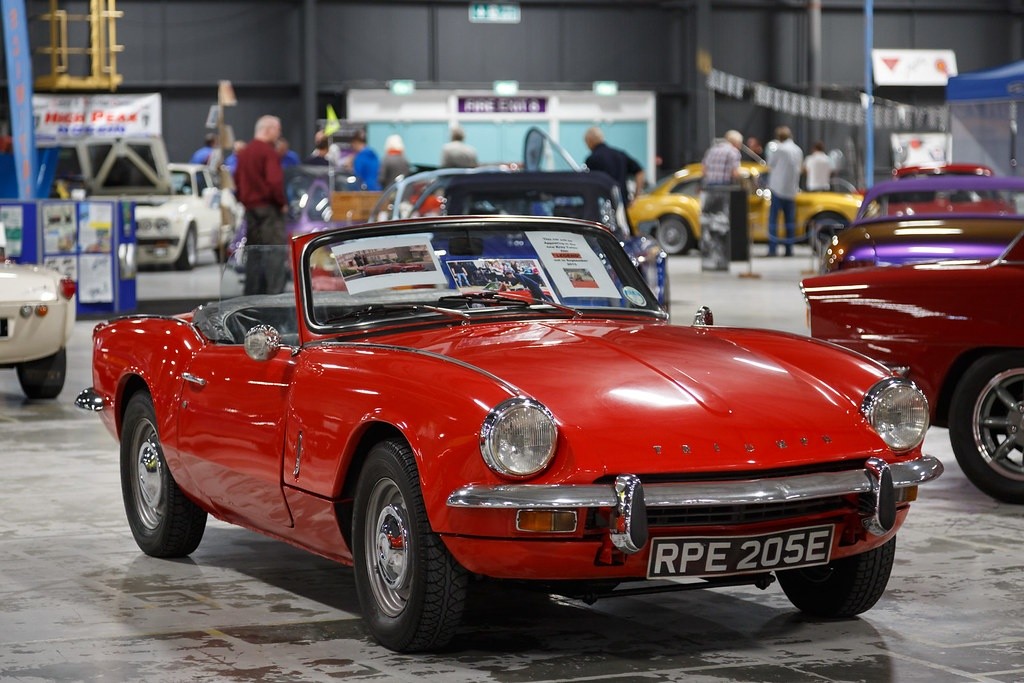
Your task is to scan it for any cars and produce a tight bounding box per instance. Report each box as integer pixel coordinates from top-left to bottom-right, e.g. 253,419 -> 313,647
0,220 -> 76,401
797,163 -> 1024,508
362,165 -> 647,306
624,160 -> 881,256
132,161 -> 230,271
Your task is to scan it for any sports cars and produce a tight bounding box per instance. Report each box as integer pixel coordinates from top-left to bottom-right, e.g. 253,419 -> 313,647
70,208 -> 946,653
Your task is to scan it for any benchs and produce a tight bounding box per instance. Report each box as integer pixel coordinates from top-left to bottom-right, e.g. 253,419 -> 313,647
200,288 -> 459,343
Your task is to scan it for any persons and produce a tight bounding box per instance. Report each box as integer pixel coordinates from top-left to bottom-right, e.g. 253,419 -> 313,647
351,132 -> 383,190
451,262 -> 471,286
187,133 -> 217,184
584,128 -> 643,203
803,140 -> 834,191
305,130 -> 341,167
743,136 -> 765,162
354,254 -> 364,267
442,128 -> 476,167
700,130 -> 743,211
379,135 -> 408,186
275,137 -> 301,198
767,126 -> 803,256
233,115 -> 289,295
484,261 -> 553,304
224,141 -> 244,172
